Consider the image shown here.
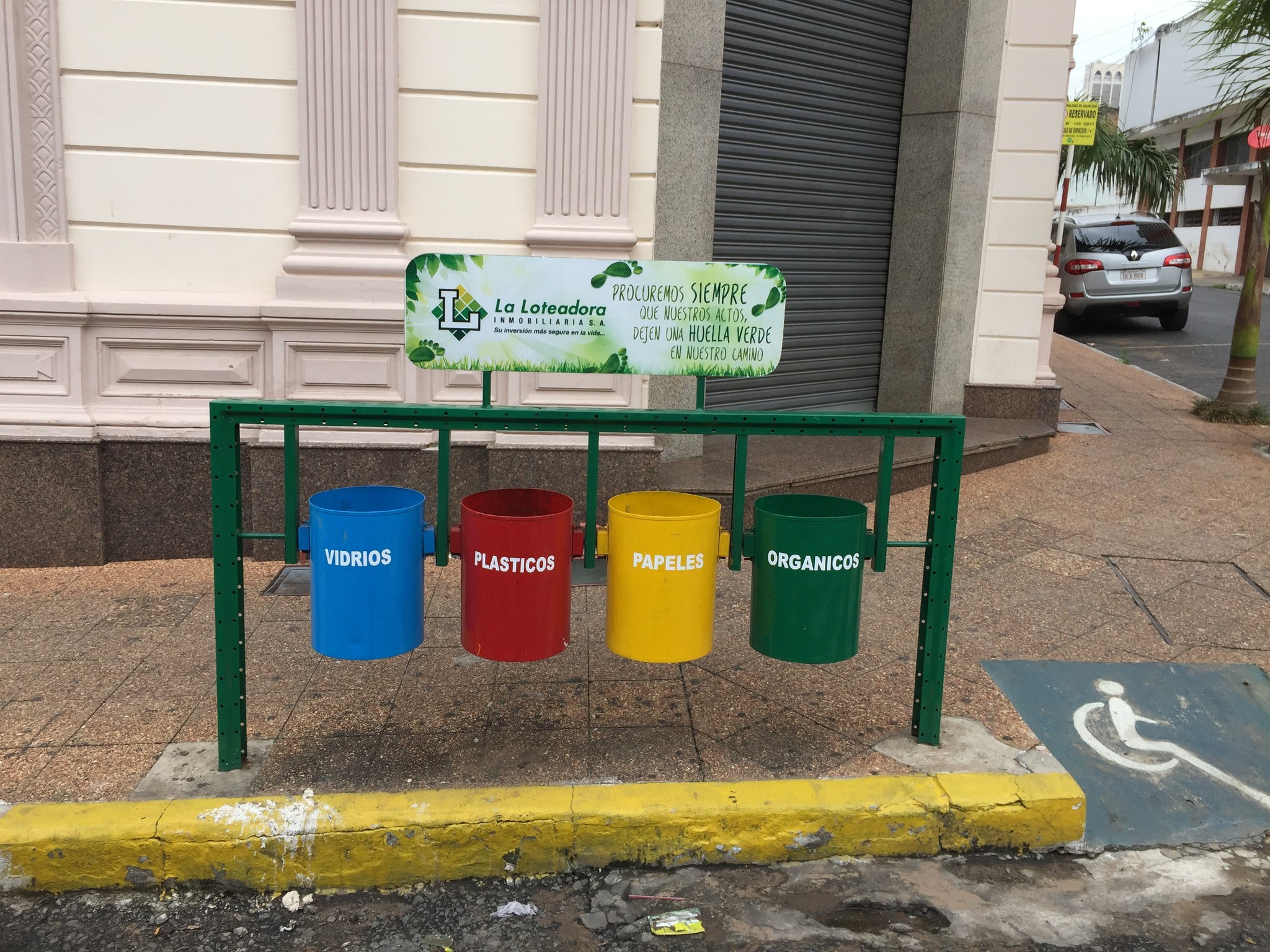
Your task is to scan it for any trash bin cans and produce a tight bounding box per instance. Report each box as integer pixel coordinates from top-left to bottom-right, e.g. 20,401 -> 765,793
744,494 -> 875,663
595,490 -> 729,664
300,484 -> 433,661
451,488 -> 584,662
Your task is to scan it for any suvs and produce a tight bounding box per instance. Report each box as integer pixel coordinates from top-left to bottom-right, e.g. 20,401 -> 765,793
1049,210 -> 1194,331
